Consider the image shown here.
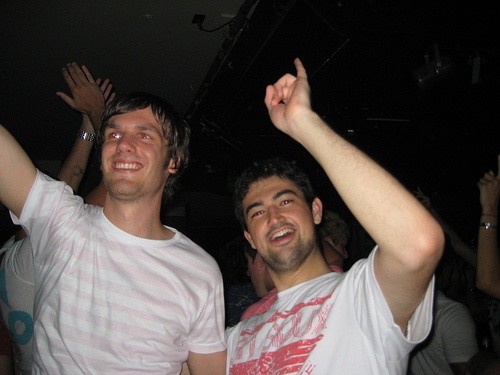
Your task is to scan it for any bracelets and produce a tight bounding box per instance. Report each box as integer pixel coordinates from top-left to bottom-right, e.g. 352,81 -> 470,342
481,213 -> 499,218
77,130 -> 96,143
480,221 -> 498,230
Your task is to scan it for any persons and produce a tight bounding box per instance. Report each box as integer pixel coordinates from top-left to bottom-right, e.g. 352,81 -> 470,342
224,152 -> 500,375
0,88 -> 228,375
224,56 -> 446,375
0,61 -> 116,375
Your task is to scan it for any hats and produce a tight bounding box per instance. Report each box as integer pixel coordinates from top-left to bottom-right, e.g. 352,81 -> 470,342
315,211 -> 350,259
